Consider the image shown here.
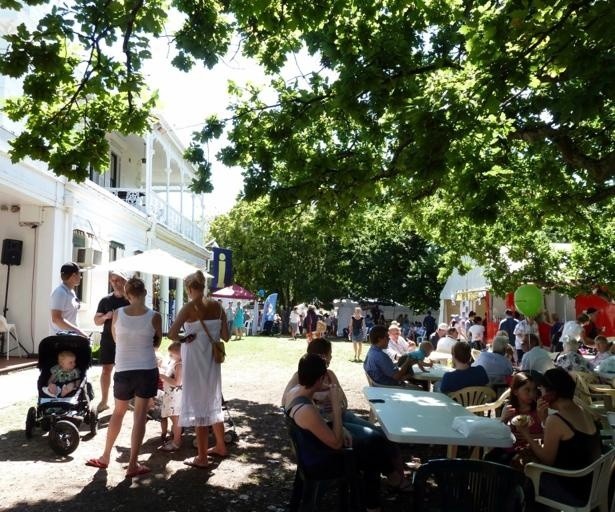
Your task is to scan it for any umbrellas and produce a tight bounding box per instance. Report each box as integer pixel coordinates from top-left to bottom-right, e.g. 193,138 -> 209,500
210,283 -> 255,299
261,293 -> 279,331
93,248 -> 215,279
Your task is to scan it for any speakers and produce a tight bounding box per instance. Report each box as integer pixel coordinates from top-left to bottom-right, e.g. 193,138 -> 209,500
0,237 -> 24,266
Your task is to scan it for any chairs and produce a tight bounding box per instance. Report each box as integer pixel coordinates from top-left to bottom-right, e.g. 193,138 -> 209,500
0,315 -> 22,360
575,372 -> 615,414
445,388 -> 515,460
409,457 -> 536,512
523,447 -> 615,512
363,369 -> 419,421
445,385 -> 497,407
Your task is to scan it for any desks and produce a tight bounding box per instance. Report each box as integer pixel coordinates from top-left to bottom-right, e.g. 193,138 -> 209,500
360,383 -> 518,461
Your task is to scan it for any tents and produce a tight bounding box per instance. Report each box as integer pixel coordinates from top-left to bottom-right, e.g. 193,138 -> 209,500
438,243 -> 615,321
333,297 -> 417,338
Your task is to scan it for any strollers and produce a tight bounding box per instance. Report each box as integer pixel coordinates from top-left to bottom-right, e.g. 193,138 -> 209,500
25,332 -> 99,457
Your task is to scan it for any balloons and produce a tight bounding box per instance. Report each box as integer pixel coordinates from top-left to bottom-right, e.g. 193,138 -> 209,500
514,283 -> 544,319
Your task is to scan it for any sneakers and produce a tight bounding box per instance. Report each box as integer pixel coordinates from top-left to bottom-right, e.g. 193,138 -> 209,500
97,401 -> 110,412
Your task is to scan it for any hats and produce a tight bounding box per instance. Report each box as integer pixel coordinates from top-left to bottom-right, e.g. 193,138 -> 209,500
438,323 -> 448,331
113,271 -> 129,281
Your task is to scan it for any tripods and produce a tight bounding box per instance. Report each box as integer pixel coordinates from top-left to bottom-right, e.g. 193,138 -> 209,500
0,265 -> 31,359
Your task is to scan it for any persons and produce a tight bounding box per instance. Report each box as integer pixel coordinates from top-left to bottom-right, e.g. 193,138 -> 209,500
583,308 -> 600,345
582,310 -> 597,339
48,262 -> 90,340
475,336 -> 513,378
365,313 -> 385,328
497,310 -> 520,360
127,379 -> 187,445
288,305 -> 338,342
167,269 -> 231,469
415,321 -> 418,324
483,311 -> 499,347
550,319 -> 570,352
468,316 -> 485,363
588,335 -> 610,369
225,301 -> 236,339
363,325 -> 423,402
281,338 -> 430,497
348,307 -> 366,362
465,311 -> 476,350
491,330 -> 513,361
556,337 -> 592,374
402,315 -> 410,341
435,327 -> 460,354
382,320 -> 417,383
445,319 -> 470,342
484,372 -> 548,465
397,314 -> 402,325
594,344 -> 615,373
512,333 -> 555,373
157,342 -> 183,451
415,322 -> 425,343
423,311 -> 436,341
284,352 -> 399,512
243,306 -> 281,336
517,367 -> 602,512
408,323 -> 416,341
556,314 -> 589,352
399,342 -> 433,376
86,278 -> 162,476
511,311 -> 521,322
513,316 -> 541,367
231,302 -> 245,340
93,269 -> 132,413
48,351 -> 81,397
371,304 -> 384,324
428,323 -> 448,350
433,342 -> 489,405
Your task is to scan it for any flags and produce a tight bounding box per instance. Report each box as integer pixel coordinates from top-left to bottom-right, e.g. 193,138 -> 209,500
212,247 -> 233,289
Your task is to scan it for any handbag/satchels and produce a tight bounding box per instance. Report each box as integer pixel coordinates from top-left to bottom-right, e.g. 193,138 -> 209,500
194,303 -> 226,365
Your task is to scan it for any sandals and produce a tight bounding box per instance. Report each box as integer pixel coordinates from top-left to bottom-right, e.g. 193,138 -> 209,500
127,463 -> 151,477
184,456 -> 209,468
86,459 -> 109,468
208,446 -> 229,457
159,440 -> 180,451
